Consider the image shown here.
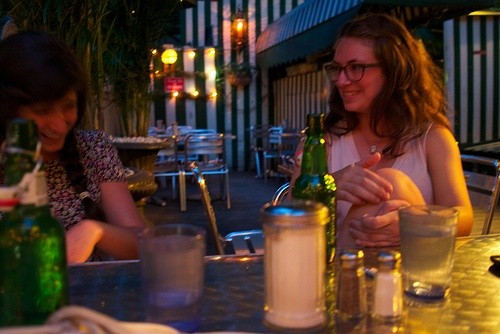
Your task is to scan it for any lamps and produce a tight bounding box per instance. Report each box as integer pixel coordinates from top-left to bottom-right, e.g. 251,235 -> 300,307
160,48 -> 178,72
230,9 -> 248,49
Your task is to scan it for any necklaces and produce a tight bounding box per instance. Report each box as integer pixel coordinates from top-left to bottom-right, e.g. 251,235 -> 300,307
358,124 -> 384,155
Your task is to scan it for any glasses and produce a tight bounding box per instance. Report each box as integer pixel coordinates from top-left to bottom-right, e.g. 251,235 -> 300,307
324,61 -> 386,81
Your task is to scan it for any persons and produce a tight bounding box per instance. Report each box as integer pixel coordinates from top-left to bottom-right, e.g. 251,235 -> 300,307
0,32 -> 147,265
289,12 -> 473,248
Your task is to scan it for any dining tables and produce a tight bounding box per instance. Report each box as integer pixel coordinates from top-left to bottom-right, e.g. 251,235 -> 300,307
67,232 -> 500,333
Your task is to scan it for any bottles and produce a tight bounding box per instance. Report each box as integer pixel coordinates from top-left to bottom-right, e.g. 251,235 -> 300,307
0,118 -> 67,329
335,249 -> 369,334
136,222 -> 206,310
291,113 -> 337,264
370,250 -> 408,334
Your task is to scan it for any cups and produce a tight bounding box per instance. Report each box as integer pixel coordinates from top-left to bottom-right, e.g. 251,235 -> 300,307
398,205 -> 459,300
258,198 -> 330,333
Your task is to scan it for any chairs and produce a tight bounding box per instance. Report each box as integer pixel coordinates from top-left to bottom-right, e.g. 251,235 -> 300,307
405,151 -> 500,235
149,123 -> 303,211
